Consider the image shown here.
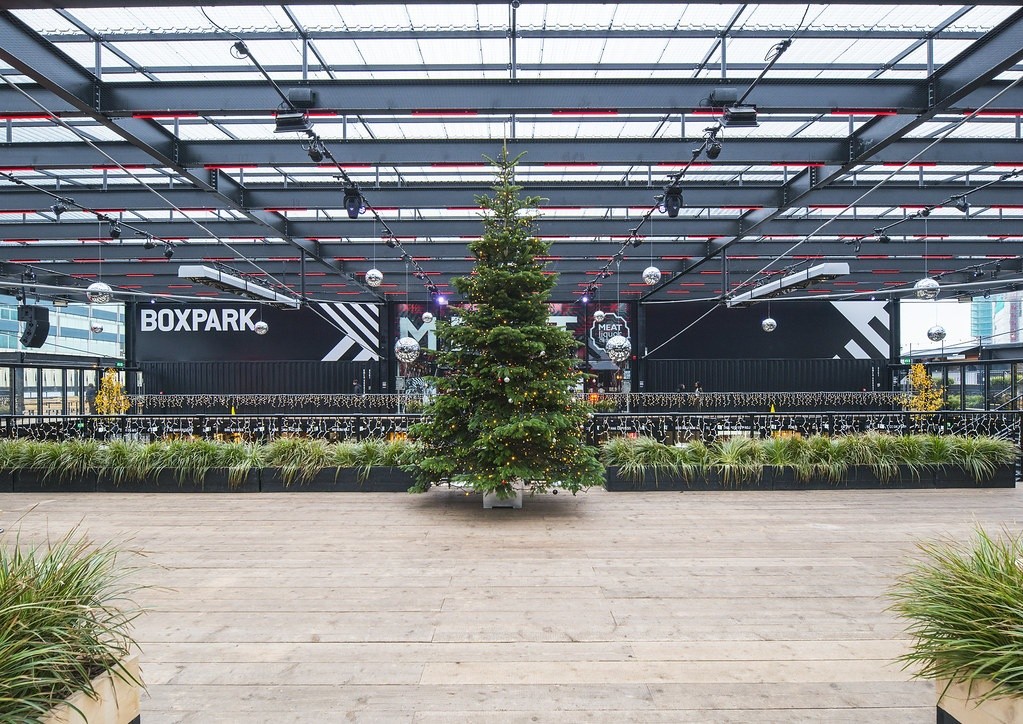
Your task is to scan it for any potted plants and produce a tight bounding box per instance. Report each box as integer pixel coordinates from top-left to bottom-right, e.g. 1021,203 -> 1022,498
0,516 -> 179,724
407,146 -> 607,509
874,513 -> 1023,724
0,435 -> 430,493
596,430 -> 1023,492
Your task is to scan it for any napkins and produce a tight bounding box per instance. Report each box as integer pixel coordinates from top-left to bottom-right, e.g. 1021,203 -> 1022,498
583,282 -> 598,302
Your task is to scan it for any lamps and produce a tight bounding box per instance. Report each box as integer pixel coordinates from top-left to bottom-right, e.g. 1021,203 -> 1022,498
705,137 -> 722,160
602,268 -> 610,279
956,196 -> 970,212
718,103 -> 760,127
163,243 -> 174,258
51,198 -> 70,215
854,242 -> 861,252
178,209 -> 300,311
878,230 -> 890,243
143,236 -> 156,249
386,235 -> 395,248
344,187 -> 366,218
416,269 -> 444,303
274,108 -> 314,133
958,263 -> 1016,303
664,186 -> 683,217
633,234 -> 642,247
308,139 -> 323,162
727,189 -> 850,309
108,222 -> 122,238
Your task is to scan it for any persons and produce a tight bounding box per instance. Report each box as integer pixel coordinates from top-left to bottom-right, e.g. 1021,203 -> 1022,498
352,378 -> 363,394
694,379 -> 703,394
86,382 -> 98,415
677,383 -> 687,393
